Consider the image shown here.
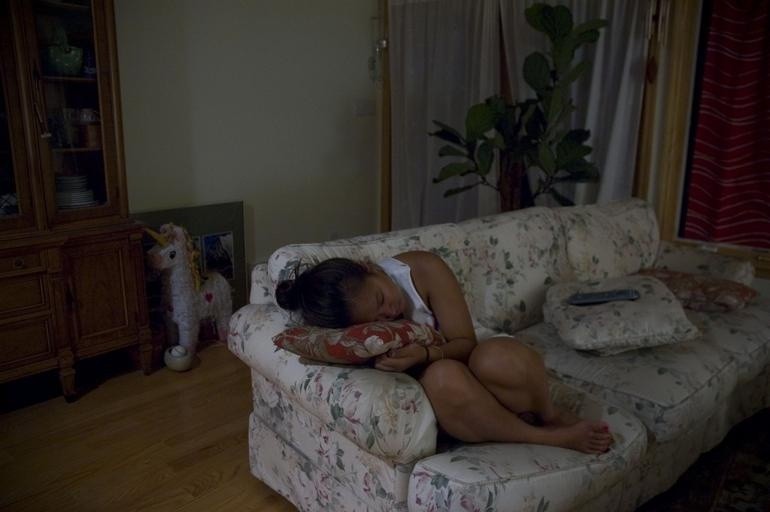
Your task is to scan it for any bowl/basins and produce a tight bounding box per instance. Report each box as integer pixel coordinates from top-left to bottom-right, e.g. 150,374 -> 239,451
42,47 -> 84,77
72,121 -> 102,149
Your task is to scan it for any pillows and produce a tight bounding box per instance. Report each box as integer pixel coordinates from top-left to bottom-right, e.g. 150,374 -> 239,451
541,271 -> 699,350
550,196 -> 669,286
255,222 -> 477,325
271,317 -> 447,365
455,205 -> 568,335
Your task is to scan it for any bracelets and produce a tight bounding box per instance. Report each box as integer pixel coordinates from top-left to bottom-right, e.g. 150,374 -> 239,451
424,346 -> 430,361
431,343 -> 445,360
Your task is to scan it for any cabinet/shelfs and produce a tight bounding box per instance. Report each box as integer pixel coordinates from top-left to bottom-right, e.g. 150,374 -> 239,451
0,0 -> 153,403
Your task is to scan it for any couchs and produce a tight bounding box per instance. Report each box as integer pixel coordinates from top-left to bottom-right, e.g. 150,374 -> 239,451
225,196 -> 767,512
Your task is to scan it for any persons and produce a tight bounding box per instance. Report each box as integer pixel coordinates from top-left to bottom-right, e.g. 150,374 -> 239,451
276,251 -> 612,455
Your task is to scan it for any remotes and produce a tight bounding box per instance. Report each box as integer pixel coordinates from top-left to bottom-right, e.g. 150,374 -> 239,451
568,289 -> 640,306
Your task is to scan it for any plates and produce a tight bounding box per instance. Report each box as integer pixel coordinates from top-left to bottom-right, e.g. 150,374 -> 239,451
54,175 -> 96,209
